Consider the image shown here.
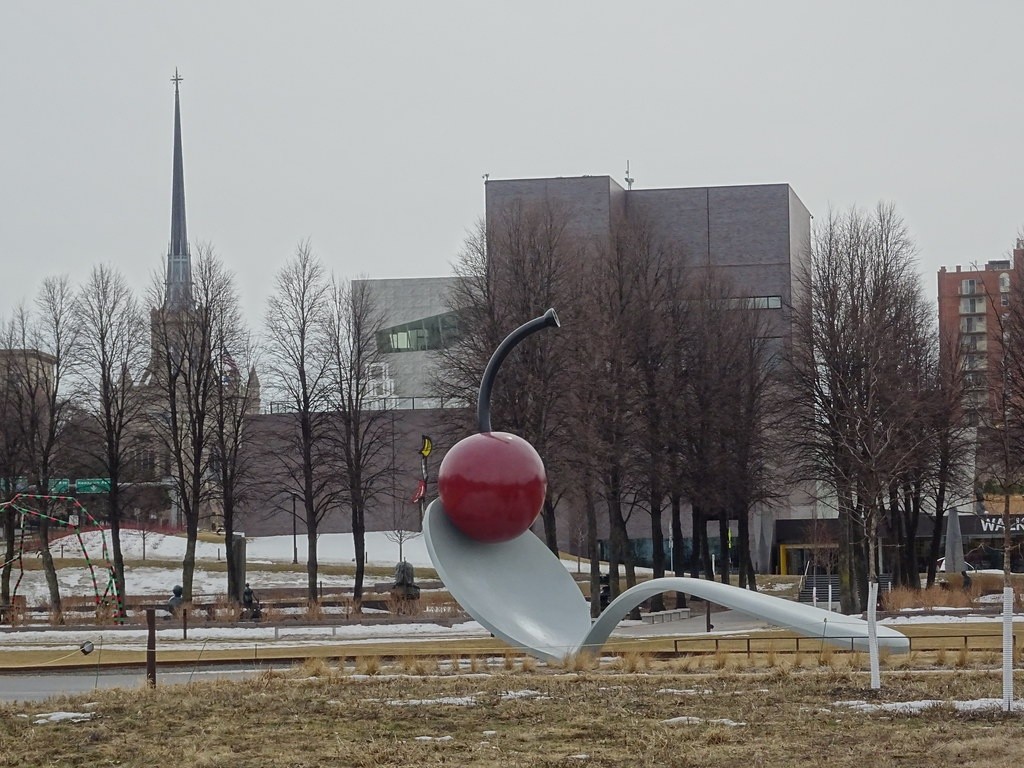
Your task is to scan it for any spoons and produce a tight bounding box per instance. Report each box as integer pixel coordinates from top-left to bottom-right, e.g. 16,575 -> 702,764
422,497 -> 909,670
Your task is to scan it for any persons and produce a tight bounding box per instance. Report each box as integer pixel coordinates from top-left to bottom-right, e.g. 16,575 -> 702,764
961,571 -> 972,590
